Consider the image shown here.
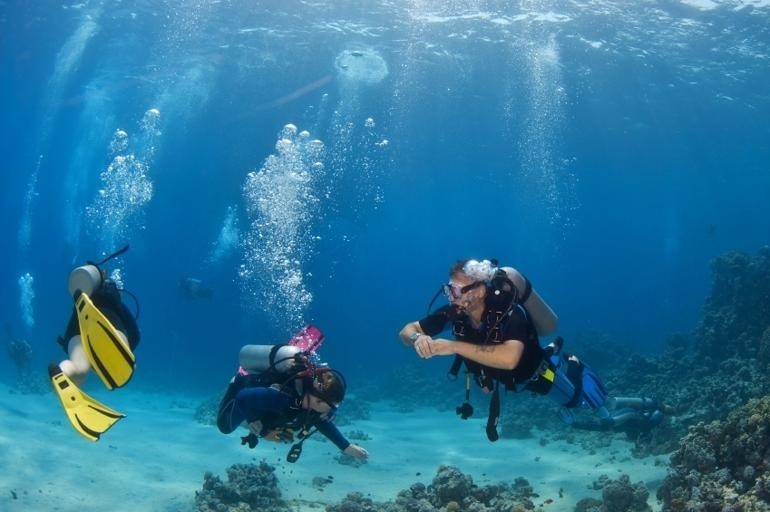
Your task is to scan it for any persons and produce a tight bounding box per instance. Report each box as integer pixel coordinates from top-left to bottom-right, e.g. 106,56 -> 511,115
46,263 -> 141,448
215,320 -> 370,461
395,256 -> 610,413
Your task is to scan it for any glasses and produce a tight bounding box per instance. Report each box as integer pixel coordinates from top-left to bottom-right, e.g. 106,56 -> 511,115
442,280 -> 484,298
322,395 -> 339,419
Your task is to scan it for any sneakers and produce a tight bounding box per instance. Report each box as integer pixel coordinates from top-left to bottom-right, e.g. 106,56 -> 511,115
552,336 -> 563,355
47,364 -> 81,406
73,288 -> 98,327
564,351 -> 584,371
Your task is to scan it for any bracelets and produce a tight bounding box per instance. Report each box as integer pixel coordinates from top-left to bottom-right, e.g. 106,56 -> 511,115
409,330 -> 420,347
